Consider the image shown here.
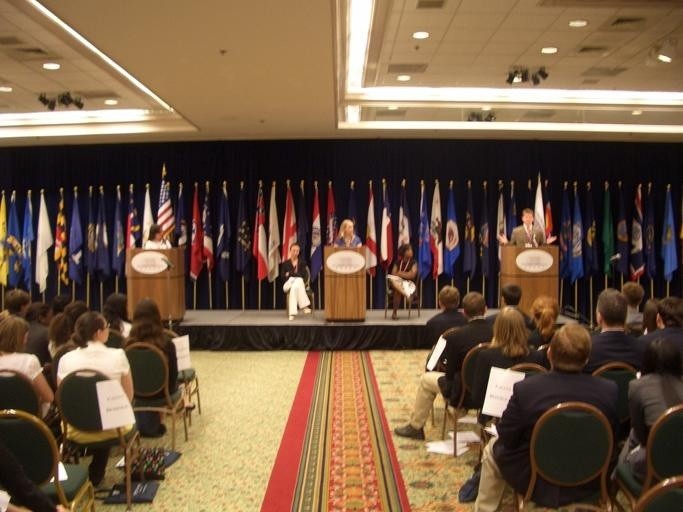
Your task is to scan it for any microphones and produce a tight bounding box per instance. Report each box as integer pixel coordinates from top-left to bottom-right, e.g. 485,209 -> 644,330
531,221 -> 534,245
161,257 -> 177,270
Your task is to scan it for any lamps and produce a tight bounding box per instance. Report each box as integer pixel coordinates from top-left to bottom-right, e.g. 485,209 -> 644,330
506,65 -> 549,85
467,110 -> 495,121
38,91 -> 86,111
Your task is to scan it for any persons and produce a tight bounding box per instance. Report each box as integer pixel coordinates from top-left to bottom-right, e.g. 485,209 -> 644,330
56,312 -> 134,487
385,244 -> 419,321
0,314 -> 63,452
1,289 -> 133,367
394,286 -> 561,440
281,244 -> 314,322
475,323 -> 621,512
122,299 -> 195,412
628,333 -> 683,489
585,283 -> 683,377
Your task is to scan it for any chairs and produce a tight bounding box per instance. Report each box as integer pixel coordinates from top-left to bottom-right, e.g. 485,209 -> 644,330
286,266 -> 314,318
431,328 -> 683,512
0,327 -> 200,512
384,271 -> 421,317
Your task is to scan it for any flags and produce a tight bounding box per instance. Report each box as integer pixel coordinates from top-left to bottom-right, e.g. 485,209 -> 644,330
1,163 -> 680,293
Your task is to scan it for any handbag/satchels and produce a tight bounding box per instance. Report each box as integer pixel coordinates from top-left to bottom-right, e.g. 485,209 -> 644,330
459,464 -> 481,502
93,448 -> 181,503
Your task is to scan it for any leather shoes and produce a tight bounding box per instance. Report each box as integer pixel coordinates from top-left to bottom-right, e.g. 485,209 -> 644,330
394,424 -> 424,440
